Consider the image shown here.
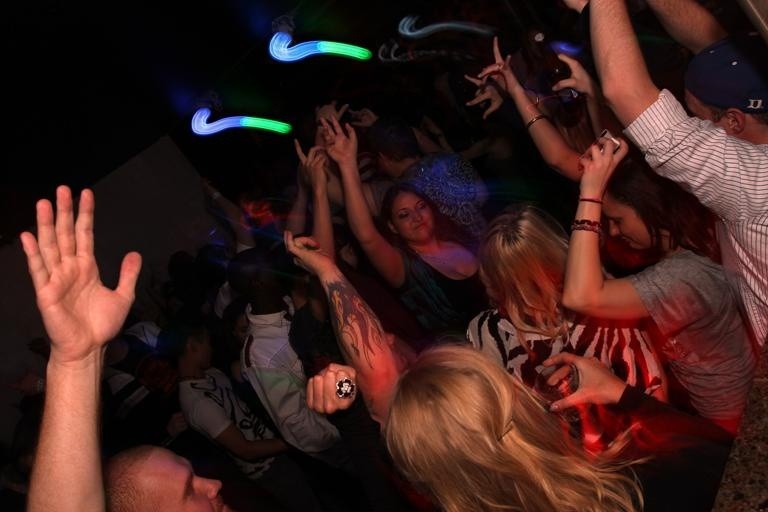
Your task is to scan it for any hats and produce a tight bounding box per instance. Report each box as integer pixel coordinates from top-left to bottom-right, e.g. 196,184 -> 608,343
685,37 -> 765,116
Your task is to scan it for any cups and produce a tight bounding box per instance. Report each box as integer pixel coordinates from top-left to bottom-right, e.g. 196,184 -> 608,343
536,364 -> 590,421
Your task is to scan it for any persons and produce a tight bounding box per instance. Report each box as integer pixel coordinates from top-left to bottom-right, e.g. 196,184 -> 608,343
0,0 -> 768,512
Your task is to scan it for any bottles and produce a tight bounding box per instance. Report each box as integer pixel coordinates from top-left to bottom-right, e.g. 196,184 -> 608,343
531,29 -> 581,104
